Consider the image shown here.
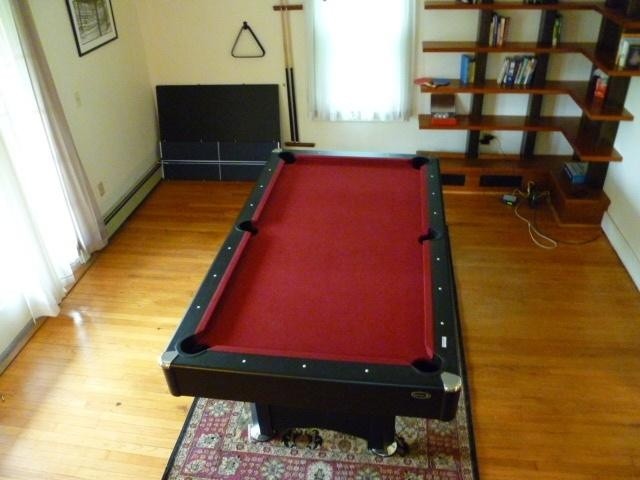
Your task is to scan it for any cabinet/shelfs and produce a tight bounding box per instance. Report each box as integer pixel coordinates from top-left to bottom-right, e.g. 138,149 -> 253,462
410,0 -> 639,230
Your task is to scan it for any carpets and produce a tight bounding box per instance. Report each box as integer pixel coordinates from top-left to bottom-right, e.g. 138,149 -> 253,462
160,376 -> 479,480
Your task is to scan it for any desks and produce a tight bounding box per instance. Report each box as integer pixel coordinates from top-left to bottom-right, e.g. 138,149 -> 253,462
155,139 -> 466,463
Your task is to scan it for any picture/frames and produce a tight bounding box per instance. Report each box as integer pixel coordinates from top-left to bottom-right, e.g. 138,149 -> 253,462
65,0 -> 120,58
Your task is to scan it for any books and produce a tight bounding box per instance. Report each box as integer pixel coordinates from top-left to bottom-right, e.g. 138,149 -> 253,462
461,0 -> 494,6
588,68 -> 607,107
413,75 -> 450,89
488,13 -> 510,48
563,160 -> 591,184
551,14 -> 562,48
430,94 -> 456,126
496,55 -> 538,88
616,32 -> 639,70
459,55 -> 476,86
523,0 -> 559,6
